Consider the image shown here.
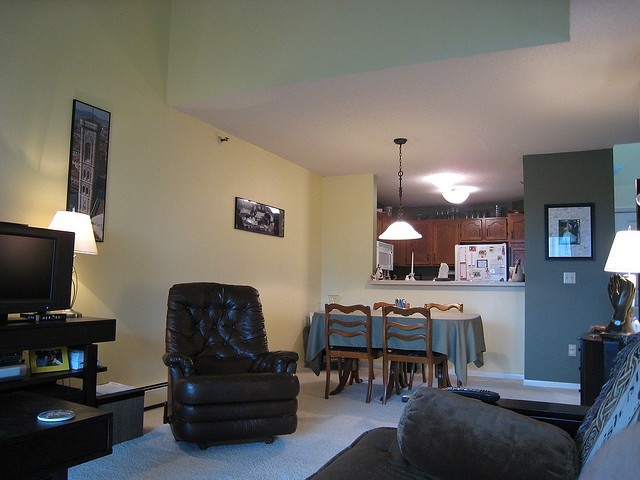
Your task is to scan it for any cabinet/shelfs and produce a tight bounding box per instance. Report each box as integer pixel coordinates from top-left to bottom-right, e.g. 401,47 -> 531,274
406,220 -> 459,264
576,332 -> 624,404
1,313 -> 116,406
460,218 -> 507,241
378,215 -> 406,265
507,214 -> 526,265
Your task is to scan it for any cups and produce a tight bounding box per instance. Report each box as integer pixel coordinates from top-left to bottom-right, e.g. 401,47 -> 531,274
434,206 -> 489,219
326,294 -> 343,303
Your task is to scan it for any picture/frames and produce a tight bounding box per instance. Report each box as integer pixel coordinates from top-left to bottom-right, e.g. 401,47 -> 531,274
235,196 -> 286,240
544,204 -> 596,262
28,347 -> 69,373
66,98 -> 111,244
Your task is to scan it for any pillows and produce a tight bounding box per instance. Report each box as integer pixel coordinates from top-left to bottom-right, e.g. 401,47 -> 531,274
392,384 -> 579,477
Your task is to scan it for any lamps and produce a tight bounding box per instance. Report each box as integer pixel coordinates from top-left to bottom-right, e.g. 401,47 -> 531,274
441,191 -> 470,205
379,140 -> 423,242
604,226 -> 640,275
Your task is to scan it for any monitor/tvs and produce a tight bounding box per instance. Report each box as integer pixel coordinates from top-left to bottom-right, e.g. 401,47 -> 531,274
0,221 -> 75,324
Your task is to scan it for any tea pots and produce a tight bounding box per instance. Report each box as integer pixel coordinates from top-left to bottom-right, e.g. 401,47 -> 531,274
513,256 -> 522,282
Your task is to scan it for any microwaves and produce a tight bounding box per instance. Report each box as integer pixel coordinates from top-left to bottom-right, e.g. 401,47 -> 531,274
376,239 -> 396,271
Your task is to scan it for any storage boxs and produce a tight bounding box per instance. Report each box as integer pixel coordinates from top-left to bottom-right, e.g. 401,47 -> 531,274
95,387 -> 145,443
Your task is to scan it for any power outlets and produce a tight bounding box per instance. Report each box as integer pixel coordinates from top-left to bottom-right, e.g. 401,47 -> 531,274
569,344 -> 576,357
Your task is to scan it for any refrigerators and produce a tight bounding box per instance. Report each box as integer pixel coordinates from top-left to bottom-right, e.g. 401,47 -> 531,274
454,244 -> 508,282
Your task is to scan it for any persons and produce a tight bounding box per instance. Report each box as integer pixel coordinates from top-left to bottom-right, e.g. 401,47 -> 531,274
561,220 -> 579,244
48,354 -> 63,365
605,274 -> 636,334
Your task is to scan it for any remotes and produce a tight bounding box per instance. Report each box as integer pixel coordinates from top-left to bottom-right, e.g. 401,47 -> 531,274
441,386 -> 500,402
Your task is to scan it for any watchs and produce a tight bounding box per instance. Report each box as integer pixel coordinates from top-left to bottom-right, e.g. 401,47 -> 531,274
611,316 -> 626,326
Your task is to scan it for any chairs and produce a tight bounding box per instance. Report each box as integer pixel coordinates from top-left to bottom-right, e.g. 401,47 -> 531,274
381,305 -> 447,403
423,302 -> 462,313
324,304 -> 380,403
373,302 -> 408,311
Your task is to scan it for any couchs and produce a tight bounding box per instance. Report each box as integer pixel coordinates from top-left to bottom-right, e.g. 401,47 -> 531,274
306,328 -> 640,480
163,282 -> 299,449
